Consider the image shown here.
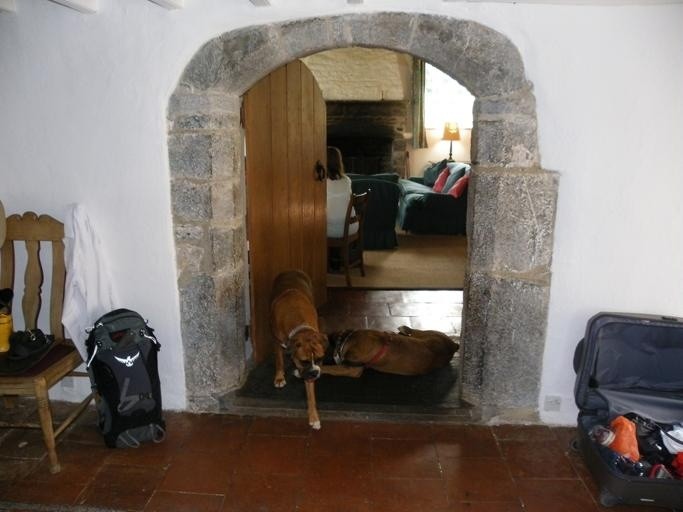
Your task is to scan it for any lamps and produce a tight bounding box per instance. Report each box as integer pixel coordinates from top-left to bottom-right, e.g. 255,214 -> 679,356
442,120 -> 460,160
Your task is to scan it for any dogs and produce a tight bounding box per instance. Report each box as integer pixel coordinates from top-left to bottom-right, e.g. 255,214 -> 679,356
269,270 -> 330,430
320,326 -> 460,378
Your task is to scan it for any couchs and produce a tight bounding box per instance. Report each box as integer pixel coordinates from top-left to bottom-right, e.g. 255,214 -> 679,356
401,156 -> 472,234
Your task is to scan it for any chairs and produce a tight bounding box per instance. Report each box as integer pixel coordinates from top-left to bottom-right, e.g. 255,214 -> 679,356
351,174 -> 402,250
327,185 -> 372,294
0,212 -> 98,479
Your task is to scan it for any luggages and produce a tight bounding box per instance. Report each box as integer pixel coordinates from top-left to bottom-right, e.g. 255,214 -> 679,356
567,310 -> 683,512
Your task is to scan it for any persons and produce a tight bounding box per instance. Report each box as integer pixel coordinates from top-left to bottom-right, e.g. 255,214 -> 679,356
326,146 -> 359,238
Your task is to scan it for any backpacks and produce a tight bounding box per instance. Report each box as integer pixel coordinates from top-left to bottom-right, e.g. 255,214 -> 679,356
84,307 -> 167,451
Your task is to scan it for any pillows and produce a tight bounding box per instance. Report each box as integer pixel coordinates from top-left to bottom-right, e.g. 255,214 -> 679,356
423,157 -> 464,198
